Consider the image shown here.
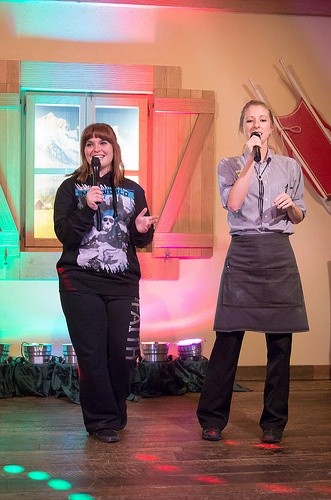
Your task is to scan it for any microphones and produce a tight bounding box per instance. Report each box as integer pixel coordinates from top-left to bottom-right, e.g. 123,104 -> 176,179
251,132 -> 261,162
91,157 -> 100,204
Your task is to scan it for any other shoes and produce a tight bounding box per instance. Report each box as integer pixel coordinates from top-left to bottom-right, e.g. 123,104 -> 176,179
89,428 -> 120,443
116,421 -> 128,431
261,428 -> 284,443
201,426 -> 224,441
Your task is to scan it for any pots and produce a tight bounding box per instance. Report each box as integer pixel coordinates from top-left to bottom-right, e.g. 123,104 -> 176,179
0,343 -> 10,363
142,341 -> 169,363
177,338 -> 202,361
61,344 -> 78,366
21,341 -> 52,365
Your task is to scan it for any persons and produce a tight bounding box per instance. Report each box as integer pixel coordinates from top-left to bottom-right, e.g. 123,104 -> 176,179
54,124 -> 159,442
195,99 -> 307,441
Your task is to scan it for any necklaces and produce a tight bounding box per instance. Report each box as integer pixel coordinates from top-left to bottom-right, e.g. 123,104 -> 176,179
255,162 -> 268,217
